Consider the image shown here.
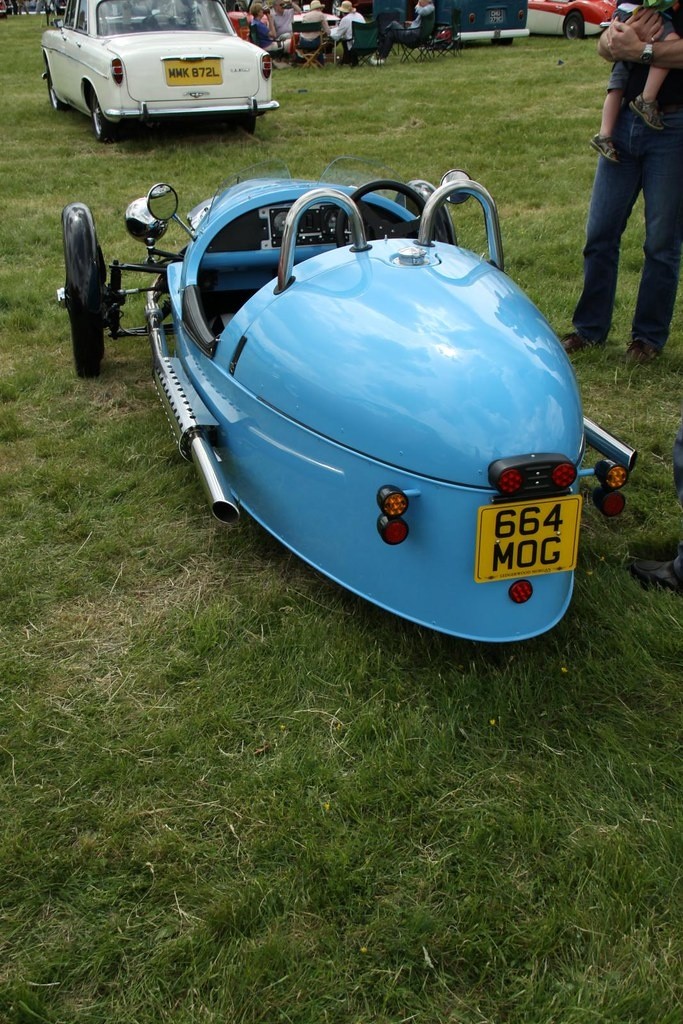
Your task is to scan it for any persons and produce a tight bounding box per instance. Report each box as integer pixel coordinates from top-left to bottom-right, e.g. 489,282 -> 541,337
559,0 -> 683,367
330,0 -> 366,68
297,0 -> 330,68
249,0 -> 300,70
370,0 -> 435,66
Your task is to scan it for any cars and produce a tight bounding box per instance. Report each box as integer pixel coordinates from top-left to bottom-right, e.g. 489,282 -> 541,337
60,156 -> 638,645
40,2 -> 281,139
526,1 -> 617,39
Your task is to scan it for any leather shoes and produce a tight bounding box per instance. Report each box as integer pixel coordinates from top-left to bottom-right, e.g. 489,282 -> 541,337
621,339 -> 662,365
560,332 -> 593,353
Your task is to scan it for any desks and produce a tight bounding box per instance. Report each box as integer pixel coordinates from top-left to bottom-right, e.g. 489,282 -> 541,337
293,13 -> 340,65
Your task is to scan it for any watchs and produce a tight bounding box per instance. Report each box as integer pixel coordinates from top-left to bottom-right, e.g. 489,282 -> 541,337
640,43 -> 654,65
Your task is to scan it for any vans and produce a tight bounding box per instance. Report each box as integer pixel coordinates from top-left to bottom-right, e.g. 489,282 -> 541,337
375,0 -> 532,45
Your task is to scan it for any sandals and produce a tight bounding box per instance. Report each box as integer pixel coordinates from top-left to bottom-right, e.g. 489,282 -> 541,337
590,133 -> 620,163
629,92 -> 664,130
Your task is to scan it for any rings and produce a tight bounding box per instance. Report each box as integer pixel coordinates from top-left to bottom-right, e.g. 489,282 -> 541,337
651,38 -> 655,42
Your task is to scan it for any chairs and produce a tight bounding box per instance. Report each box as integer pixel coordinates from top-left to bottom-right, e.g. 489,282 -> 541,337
249,8 -> 462,69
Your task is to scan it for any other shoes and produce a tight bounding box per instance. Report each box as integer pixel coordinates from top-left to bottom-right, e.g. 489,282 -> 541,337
371,58 -> 384,65
629,559 -> 683,596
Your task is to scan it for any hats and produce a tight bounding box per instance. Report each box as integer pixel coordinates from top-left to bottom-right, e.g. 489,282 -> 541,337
308,0 -> 325,10
336,1 -> 356,13
